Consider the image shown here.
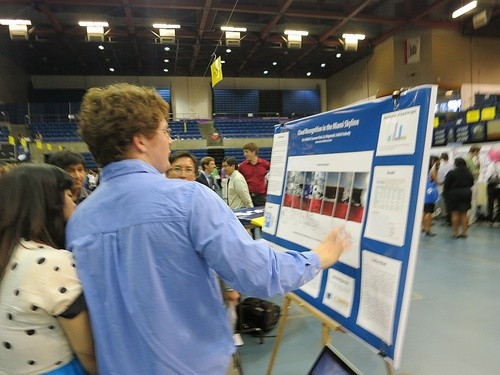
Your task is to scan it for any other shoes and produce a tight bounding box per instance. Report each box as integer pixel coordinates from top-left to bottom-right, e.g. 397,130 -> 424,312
451,235 -> 458,240
459,235 -> 467,239
423,229 -> 436,237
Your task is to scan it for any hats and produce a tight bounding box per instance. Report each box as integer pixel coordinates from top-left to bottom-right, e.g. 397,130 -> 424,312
487,148 -> 500,162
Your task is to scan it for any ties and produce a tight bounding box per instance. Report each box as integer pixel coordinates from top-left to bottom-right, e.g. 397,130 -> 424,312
208,177 -> 212,187
227,178 -> 231,206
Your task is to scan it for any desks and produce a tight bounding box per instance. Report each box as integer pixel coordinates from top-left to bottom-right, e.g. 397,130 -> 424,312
233,206 -> 265,241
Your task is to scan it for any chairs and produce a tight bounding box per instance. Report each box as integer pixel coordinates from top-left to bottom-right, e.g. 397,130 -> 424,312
37,115 -> 280,169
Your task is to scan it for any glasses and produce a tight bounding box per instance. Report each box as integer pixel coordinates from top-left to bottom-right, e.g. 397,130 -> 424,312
140,124 -> 171,137
168,165 -> 196,174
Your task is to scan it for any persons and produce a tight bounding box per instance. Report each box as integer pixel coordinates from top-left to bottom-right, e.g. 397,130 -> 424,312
426,145 -> 499,230
422,155 -> 441,236
83,167 -> 103,190
194,156 -> 216,191
238,141 -> 270,242
48,148 -> 96,201
442,157 -> 473,239
167,151 -> 198,183
67,81 -> 352,375
221,155 -> 257,225
0,161 -> 100,375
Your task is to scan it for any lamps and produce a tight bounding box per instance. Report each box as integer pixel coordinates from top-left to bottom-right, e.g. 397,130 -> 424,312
219,23 -> 247,34
343,32 -> 366,39
452,1 -> 478,19
78,19 -> 109,28
284,28 -> 309,35
0,17 -> 33,26
153,21 -> 181,29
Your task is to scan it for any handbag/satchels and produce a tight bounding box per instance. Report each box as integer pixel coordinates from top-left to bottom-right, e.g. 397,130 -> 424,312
424,169 -> 439,204
235,297 -> 280,336
487,163 -> 500,190
